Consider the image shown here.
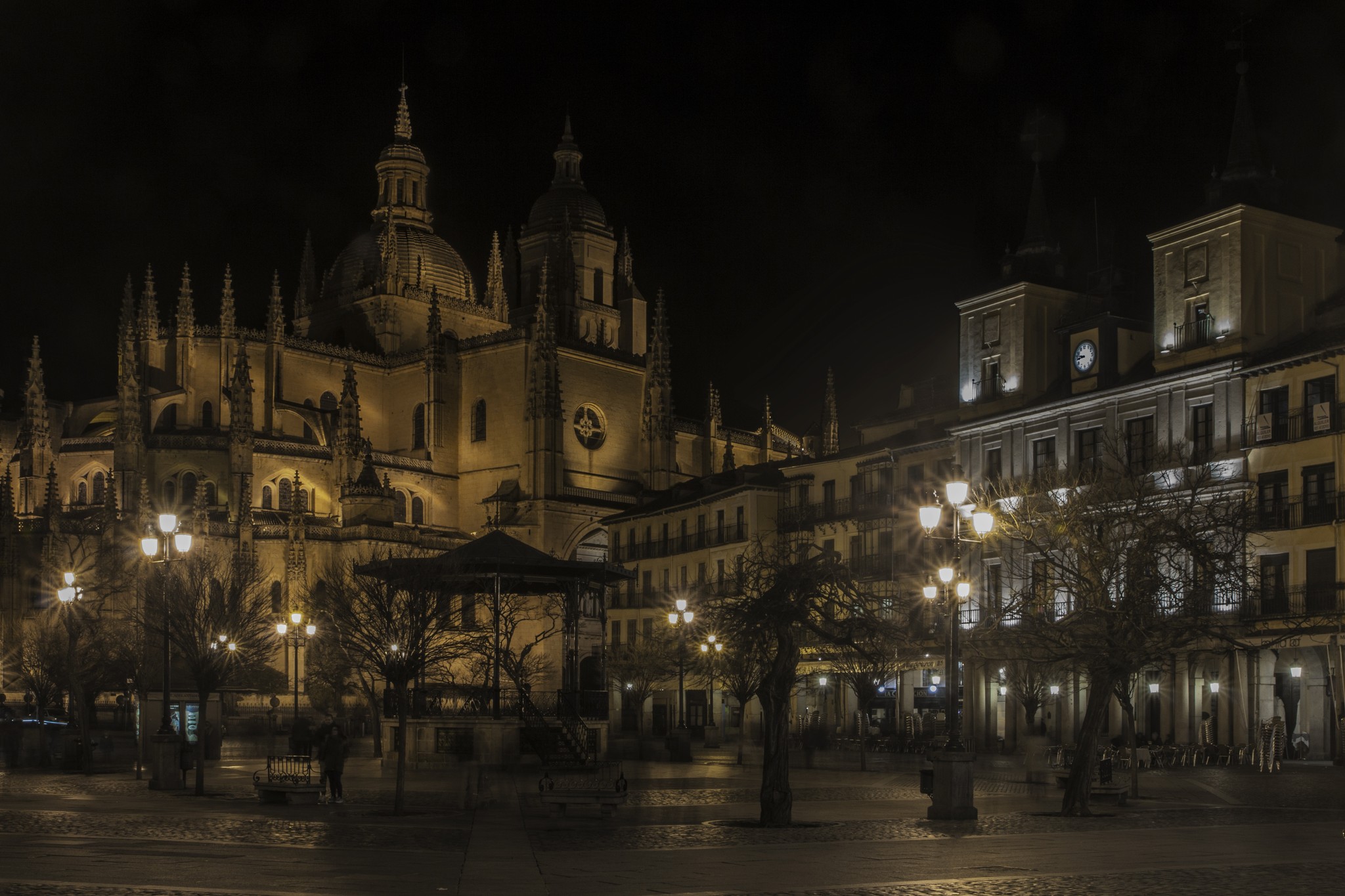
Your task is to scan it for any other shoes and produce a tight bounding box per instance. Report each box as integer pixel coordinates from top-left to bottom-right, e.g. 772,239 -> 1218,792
318,795 -> 325,802
335,797 -> 344,803
328,797 -> 337,803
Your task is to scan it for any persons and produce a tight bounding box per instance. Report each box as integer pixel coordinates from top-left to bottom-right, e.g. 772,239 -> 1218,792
287,714 -> 349,803
1149,732 -> 1163,766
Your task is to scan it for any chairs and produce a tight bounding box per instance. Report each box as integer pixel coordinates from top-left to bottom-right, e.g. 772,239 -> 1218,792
1023,717 -> 1285,773
799,707 -> 938,755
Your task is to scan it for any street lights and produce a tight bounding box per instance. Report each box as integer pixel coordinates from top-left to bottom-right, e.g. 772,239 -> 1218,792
136,512 -> 193,790
700,634 -> 724,748
276,611 -> 317,759
209,635 -> 235,715
668,599 -> 695,761
915,481 -> 994,822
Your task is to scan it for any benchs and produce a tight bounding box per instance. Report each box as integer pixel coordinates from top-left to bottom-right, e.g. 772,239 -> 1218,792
253,755 -> 326,805
538,759 -> 628,819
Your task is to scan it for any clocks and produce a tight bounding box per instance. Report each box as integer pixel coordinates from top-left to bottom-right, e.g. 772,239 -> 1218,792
1073,339 -> 1097,374
